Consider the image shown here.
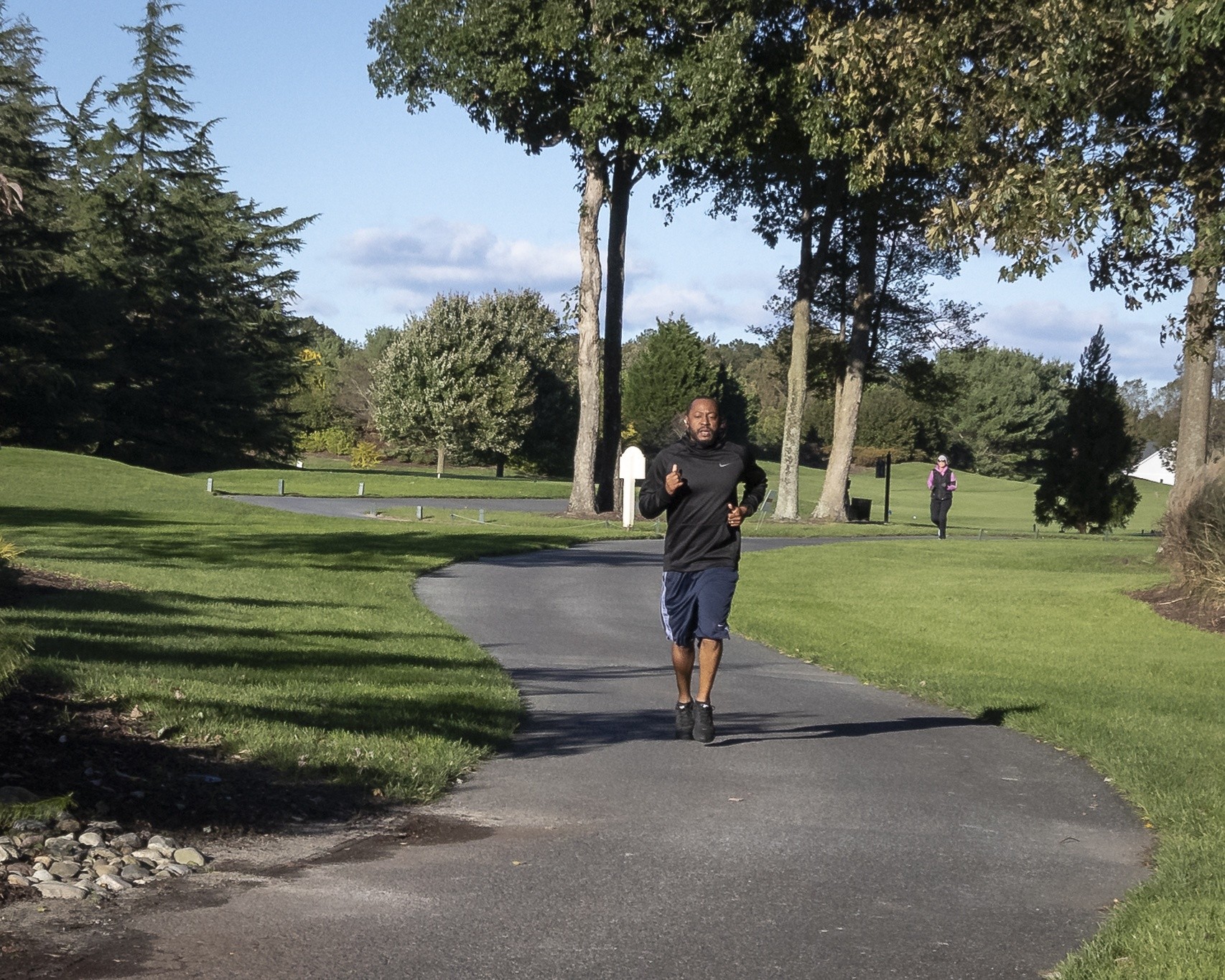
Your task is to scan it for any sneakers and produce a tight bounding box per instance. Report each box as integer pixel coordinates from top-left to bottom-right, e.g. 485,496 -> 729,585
675,696 -> 695,735
692,698 -> 716,743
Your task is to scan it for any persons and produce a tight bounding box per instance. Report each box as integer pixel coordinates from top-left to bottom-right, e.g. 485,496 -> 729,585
927,454 -> 957,540
638,395 -> 768,744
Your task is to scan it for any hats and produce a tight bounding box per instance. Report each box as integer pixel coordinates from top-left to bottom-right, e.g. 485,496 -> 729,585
937,455 -> 948,465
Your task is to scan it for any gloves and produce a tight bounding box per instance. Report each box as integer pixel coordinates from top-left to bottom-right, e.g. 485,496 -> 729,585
930,485 -> 935,489
942,483 -> 948,489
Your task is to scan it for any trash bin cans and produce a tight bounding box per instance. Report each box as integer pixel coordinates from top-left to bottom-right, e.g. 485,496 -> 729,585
850,498 -> 872,520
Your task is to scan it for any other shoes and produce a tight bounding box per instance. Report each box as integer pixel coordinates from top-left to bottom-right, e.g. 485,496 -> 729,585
937,529 -> 940,536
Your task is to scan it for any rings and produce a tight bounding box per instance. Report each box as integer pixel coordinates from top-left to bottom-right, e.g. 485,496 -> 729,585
730,521 -> 733,524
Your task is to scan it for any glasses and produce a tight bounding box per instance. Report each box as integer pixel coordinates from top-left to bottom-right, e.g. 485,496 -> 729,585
938,459 -> 945,462
